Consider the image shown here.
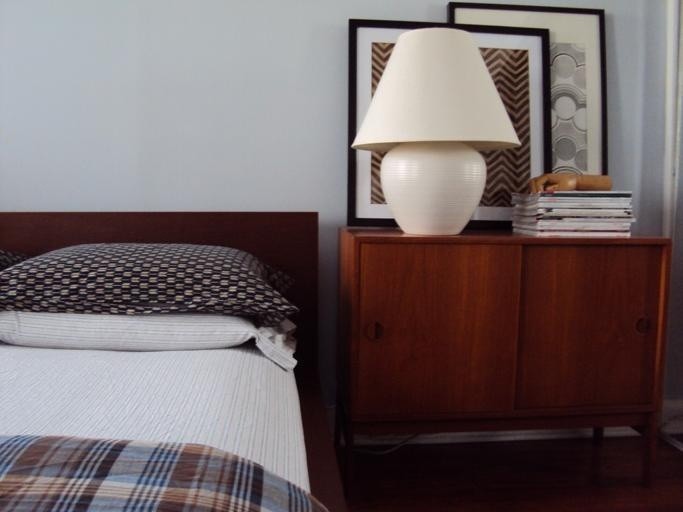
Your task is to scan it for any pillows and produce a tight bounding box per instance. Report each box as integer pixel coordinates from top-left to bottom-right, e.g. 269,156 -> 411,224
1,243 -> 299,328
0,248 -> 27,272
0,311 -> 300,371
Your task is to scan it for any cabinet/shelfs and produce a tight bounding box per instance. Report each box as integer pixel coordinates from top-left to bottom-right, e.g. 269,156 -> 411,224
336,224 -> 672,494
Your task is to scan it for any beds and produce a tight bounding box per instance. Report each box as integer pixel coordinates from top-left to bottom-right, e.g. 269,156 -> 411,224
0,211 -> 328,512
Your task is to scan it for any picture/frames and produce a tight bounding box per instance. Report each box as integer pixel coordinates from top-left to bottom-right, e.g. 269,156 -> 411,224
446,3 -> 607,182
347,17 -> 550,225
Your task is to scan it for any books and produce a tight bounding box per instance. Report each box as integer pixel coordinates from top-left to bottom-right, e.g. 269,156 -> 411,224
512,190 -> 636,237
